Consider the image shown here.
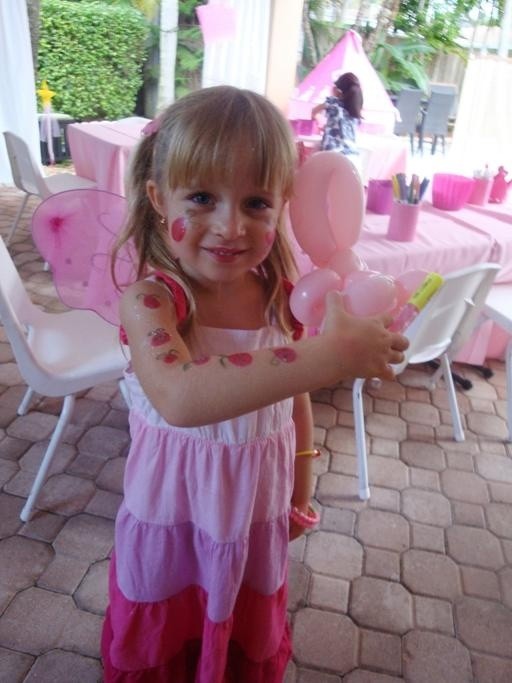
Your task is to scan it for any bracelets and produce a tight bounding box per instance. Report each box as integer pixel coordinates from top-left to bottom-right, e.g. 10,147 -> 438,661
288,502 -> 320,529
294,448 -> 321,457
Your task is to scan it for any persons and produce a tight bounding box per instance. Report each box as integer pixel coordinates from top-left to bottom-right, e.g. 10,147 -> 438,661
311,73 -> 368,175
101,85 -> 409,683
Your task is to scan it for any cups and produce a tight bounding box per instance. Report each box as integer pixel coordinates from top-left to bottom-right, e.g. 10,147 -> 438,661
365,165 -> 512,243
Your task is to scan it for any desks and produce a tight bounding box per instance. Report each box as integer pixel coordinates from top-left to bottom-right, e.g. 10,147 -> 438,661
67,116 -> 408,197
282,187 -> 512,366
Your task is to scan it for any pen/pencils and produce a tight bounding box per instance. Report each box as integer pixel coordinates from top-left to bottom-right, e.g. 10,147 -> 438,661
391,173 -> 429,205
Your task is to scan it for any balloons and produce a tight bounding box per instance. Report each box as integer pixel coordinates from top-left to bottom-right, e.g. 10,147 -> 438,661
288,150 -> 430,344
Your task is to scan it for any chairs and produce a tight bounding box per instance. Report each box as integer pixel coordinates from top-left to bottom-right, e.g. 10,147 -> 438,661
0,235 -> 131,524
428,283 -> 511,439
418,92 -> 455,156
394,87 -> 423,156
3,131 -> 97,273
353,262 -> 501,500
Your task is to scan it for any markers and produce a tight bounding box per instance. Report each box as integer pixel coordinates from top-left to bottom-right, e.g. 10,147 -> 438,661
388,273 -> 444,335
